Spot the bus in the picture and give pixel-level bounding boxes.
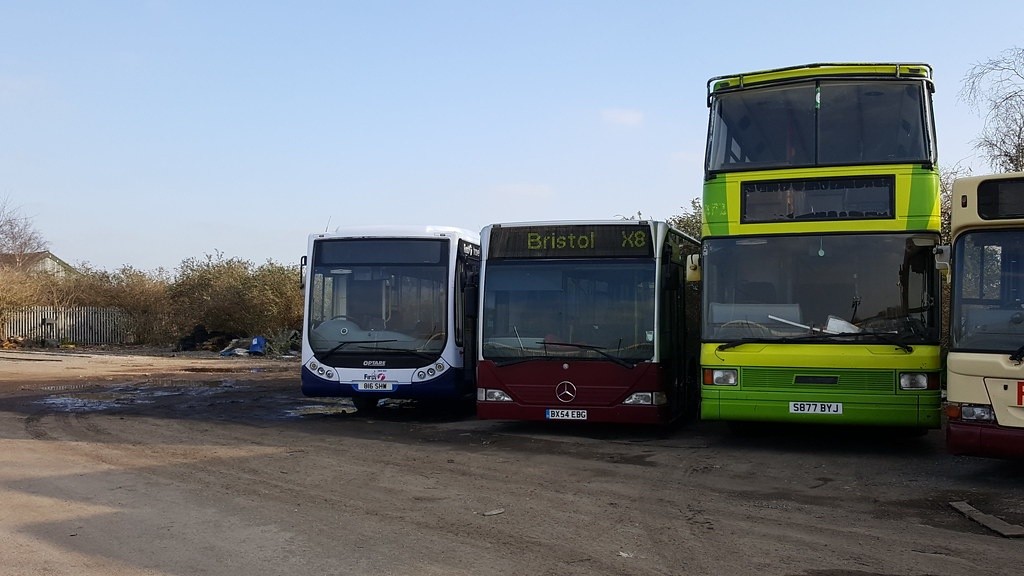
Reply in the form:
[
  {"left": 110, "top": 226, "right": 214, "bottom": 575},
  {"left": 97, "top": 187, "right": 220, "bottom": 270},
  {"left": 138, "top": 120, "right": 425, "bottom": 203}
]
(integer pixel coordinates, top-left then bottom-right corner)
[
  {"left": 686, "top": 61, "right": 952, "bottom": 436},
  {"left": 298, "top": 224, "right": 482, "bottom": 414},
  {"left": 463, "top": 218, "right": 715, "bottom": 428},
  {"left": 933, "top": 171, "right": 1024, "bottom": 459}
]
